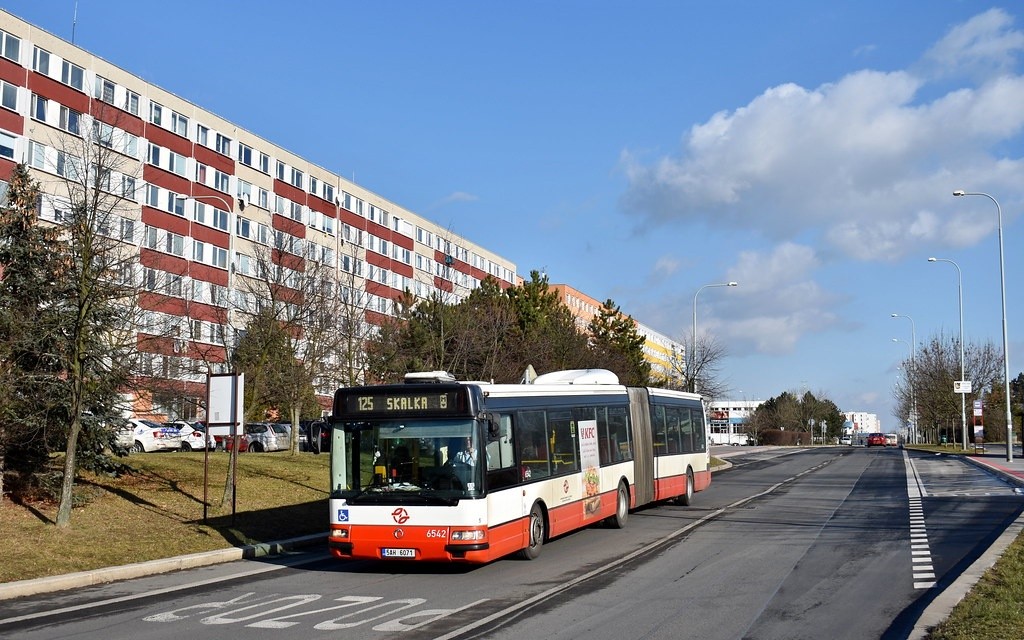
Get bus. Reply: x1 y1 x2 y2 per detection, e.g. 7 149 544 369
307 365 712 564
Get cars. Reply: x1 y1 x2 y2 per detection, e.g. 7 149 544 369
278 422 329 452
866 433 886 447
82 411 136 450
840 437 852 446
884 433 898 445
130 419 184 454
191 421 249 453
244 423 290 453
160 419 217 453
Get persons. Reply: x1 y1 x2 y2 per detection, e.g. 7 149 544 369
454 438 477 466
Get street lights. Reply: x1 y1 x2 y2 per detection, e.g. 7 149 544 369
926 257 969 454
951 189 1013 463
174 188 235 373
891 312 920 447
692 281 740 394
